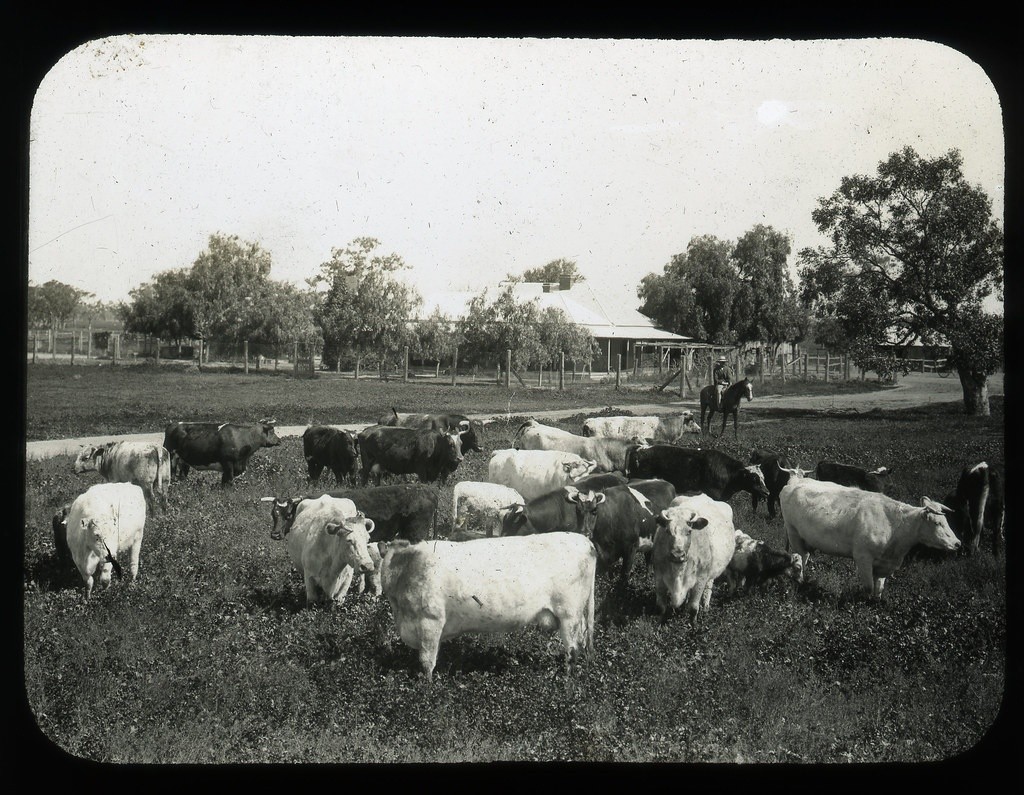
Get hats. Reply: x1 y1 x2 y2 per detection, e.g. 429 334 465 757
715 355 729 362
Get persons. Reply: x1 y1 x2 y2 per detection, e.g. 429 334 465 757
713 355 733 411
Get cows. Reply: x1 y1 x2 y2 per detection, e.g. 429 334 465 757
163 414 282 488
260 409 1002 684
52 481 147 602
73 441 172 516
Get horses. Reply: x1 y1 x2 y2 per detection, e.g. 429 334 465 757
700 376 755 439
